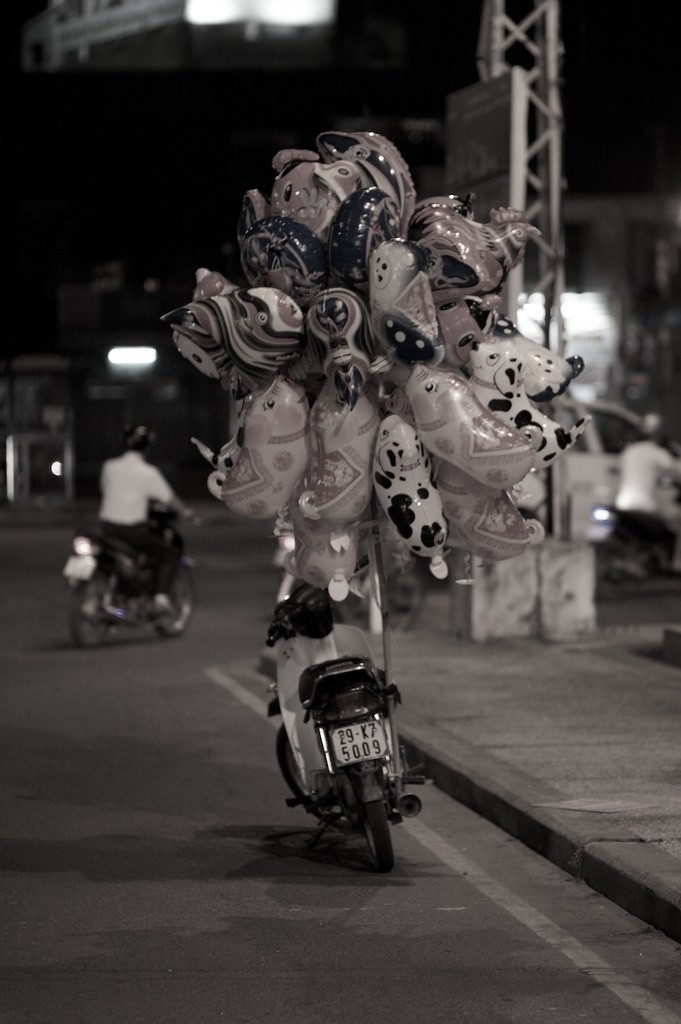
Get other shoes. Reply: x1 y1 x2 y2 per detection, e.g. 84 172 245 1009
154 594 174 612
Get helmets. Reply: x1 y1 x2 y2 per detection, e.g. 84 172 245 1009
641 413 672 439
124 423 158 451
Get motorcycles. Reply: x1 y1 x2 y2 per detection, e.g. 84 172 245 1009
584 503 681 600
266 554 426 873
62 502 197 649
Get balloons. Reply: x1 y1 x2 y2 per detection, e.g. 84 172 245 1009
161 130 594 589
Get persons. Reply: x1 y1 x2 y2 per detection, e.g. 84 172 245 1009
96 427 198 619
609 412 681 573
